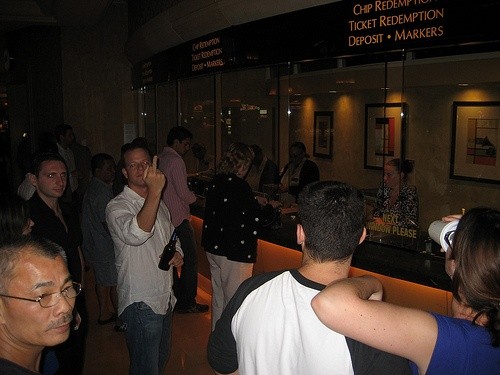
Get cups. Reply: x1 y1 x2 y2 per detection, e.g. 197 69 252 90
283 194 292 208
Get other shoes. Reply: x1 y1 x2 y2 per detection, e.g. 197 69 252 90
115 322 126 332
176 304 209 315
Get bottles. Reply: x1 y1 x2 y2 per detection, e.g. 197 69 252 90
157 229 181 271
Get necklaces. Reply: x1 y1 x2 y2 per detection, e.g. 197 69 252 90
472 310 485 324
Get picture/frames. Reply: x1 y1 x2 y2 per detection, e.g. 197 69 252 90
314 110 333 157
363 102 408 171
448 100 500 184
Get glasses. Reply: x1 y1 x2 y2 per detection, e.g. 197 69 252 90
0 280 81 308
444 230 458 253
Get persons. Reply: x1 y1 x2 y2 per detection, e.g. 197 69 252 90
277 143 318 205
105 145 184 375
200 143 280 331
207 180 411 375
191 144 215 176
153 127 208 314
0 194 60 375
24 154 89 375
369 158 419 231
7 124 154 332
244 147 280 195
0 235 82 375
311 206 500 375
